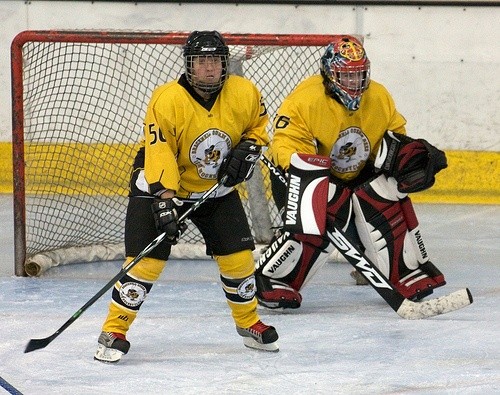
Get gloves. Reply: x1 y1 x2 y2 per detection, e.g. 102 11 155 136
151 198 187 245
218 137 260 187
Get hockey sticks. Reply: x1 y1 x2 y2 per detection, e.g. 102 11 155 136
23 174 228 354
259 156 473 319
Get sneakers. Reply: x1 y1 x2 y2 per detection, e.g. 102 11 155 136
94 331 130 363
235 320 279 352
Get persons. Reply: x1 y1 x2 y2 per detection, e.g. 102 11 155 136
93 31 280 364
254 37 447 311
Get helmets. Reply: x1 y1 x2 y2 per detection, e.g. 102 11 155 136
184 30 230 94
320 38 370 110
